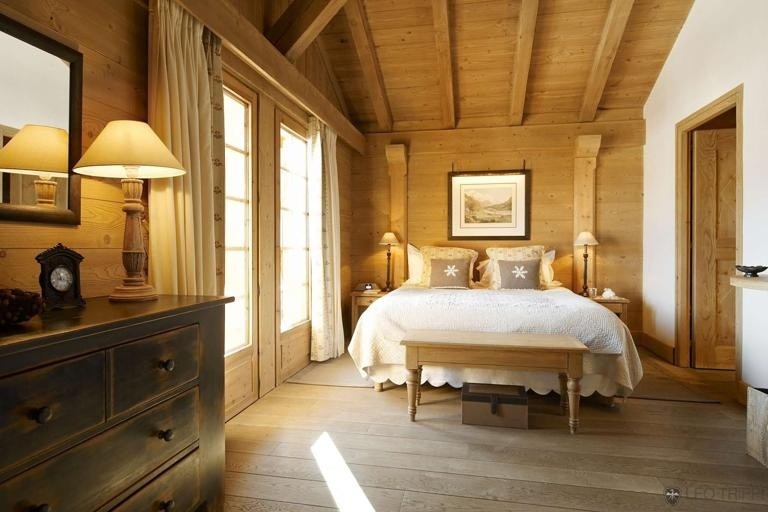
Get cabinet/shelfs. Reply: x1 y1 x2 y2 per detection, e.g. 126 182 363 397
1 296 234 511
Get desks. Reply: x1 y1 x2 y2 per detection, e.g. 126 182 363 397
399 330 590 434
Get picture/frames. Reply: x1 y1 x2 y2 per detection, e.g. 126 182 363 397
447 168 531 241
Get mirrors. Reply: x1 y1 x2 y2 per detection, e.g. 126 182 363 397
0 14 81 226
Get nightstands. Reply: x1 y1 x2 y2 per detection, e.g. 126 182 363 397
592 298 630 324
349 292 388 335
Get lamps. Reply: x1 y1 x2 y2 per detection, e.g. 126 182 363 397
574 233 599 296
0 126 70 208
379 233 400 293
73 120 189 303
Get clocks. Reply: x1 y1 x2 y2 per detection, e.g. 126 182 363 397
36 243 84 306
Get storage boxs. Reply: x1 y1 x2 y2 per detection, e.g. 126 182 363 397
462 383 529 430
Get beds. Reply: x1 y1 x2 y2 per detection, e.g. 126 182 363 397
348 243 643 403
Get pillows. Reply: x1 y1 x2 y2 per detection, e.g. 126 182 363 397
405 243 423 286
476 250 561 287
486 246 546 288
498 260 541 288
421 245 478 290
429 256 471 289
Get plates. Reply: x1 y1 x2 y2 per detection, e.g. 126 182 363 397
736 265 768 278
